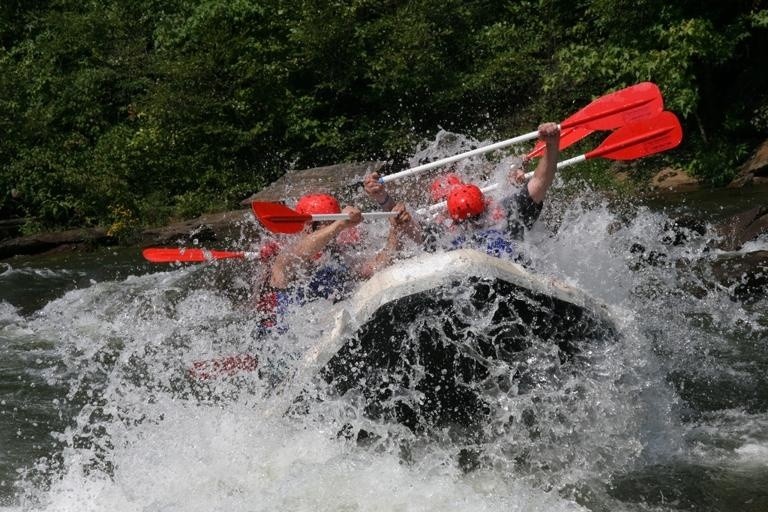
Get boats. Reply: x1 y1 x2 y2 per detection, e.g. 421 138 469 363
253 247 630 477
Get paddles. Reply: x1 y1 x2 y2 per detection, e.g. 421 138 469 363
251 200 401 234
142 248 419 263
479 124 594 189
378 81 663 185
413 111 682 217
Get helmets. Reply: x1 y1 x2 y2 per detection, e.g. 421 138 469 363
295 194 343 233
430 174 460 203
447 185 483 223
